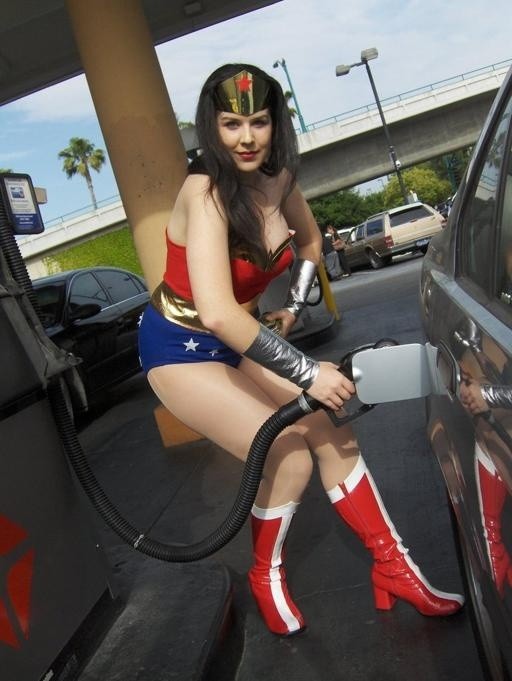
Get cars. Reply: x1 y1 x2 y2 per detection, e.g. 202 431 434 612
419 65 512 681
312 202 447 279
30 266 152 393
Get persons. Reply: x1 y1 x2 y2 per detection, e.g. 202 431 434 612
322 234 345 281
327 225 351 277
461 380 512 601
137 63 466 636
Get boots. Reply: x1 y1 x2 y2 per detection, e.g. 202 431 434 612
474 443 512 601
325 447 465 617
247 502 305 637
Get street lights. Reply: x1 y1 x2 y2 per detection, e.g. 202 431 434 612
335 48 410 203
272 58 308 134
